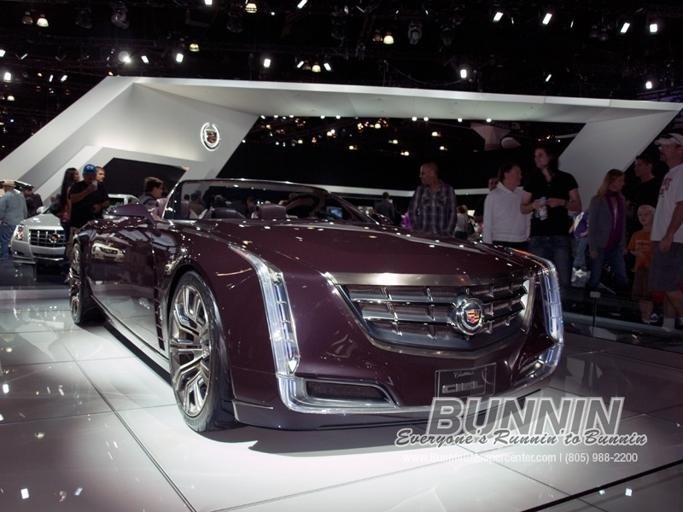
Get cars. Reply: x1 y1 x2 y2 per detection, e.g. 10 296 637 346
10 193 142 274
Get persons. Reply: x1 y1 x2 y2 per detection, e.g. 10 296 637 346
0 165 258 283
375 131 682 338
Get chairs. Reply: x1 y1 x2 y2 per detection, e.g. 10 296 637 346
152 197 288 219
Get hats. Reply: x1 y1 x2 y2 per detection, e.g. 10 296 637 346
82 163 96 176
655 131 682 146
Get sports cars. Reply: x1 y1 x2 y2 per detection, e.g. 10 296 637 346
64 176 568 438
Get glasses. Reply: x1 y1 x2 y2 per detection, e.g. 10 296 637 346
659 133 681 143
